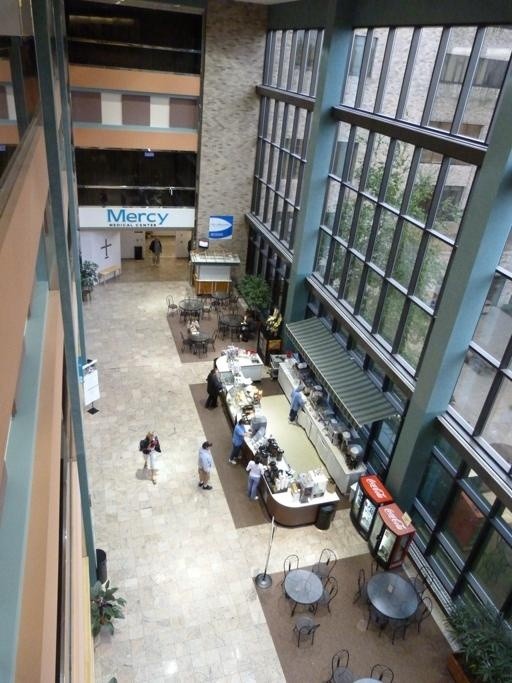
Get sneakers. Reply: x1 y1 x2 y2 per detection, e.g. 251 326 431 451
251 495 259 501
199 480 212 490
228 455 242 464
141 463 158 485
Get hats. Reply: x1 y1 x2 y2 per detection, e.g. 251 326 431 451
238 416 248 425
298 385 306 391
202 441 213 448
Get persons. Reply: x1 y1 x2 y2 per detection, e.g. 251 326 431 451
288 384 307 424
138 430 162 483
203 368 222 410
245 454 264 500
148 235 162 267
229 417 250 464
198 440 213 489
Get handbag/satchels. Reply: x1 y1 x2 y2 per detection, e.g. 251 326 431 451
140 437 162 454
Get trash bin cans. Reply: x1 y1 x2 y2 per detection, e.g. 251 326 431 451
315 503 334 530
135 247 142 259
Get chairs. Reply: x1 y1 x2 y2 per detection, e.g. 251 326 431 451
280 548 434 683
166 283 247 359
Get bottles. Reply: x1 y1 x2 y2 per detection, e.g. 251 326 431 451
291 482 298 500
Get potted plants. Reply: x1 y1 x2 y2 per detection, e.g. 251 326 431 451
90 579 125 637
237 275 273 334
443 595 512 683
80 259 99 302
